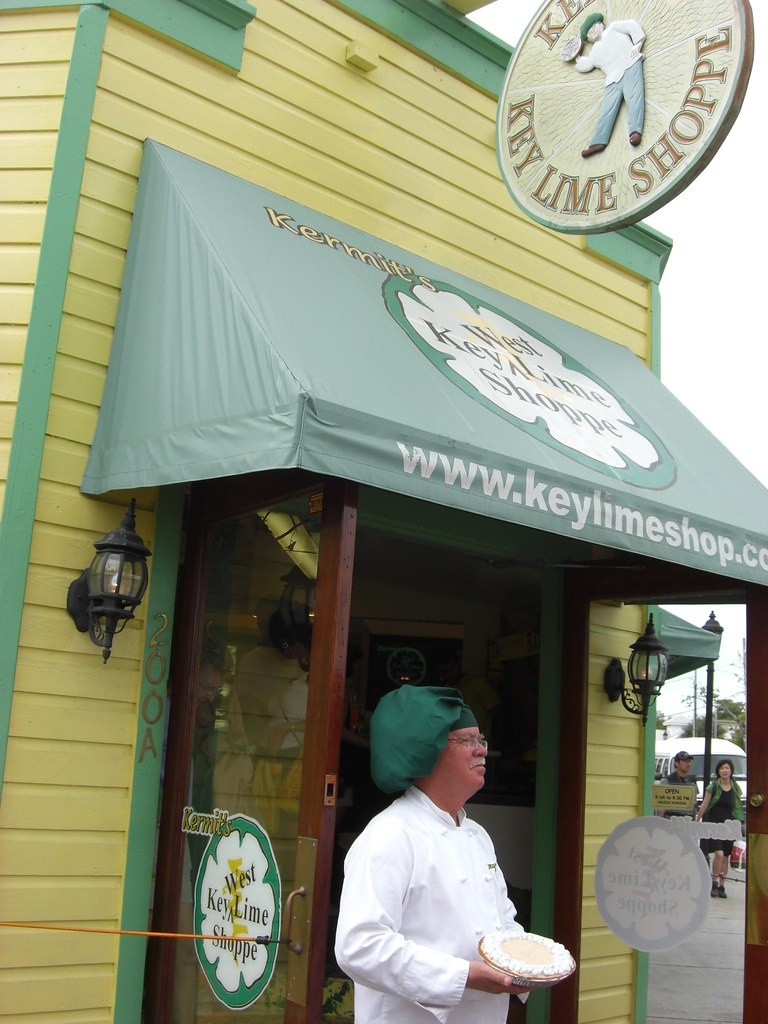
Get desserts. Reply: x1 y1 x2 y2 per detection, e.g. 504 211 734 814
481 931 574 978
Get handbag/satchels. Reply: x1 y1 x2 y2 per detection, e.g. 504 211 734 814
729 840 746 873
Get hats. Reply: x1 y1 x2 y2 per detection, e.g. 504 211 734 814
370 684 479 792
675 751 694 760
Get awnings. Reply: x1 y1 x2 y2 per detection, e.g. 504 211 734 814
78 137 768 606
659 606 721 680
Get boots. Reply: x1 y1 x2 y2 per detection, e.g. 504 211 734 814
710 881 719 896
718 886 727 898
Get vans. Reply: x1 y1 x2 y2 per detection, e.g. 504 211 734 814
653 736 747 823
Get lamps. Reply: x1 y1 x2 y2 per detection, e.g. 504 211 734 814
269 560 316 684
602 612 665 728
65 498 151 663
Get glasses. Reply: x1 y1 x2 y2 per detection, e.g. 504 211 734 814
447 733 488 754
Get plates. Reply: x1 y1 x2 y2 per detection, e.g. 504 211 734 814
479 954 576 988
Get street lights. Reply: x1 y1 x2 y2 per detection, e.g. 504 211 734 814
700 611 726 804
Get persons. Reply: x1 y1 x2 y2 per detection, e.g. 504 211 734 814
574 13 647 158
658 751 701 822
697 759 745 898
334 684 538 1024
